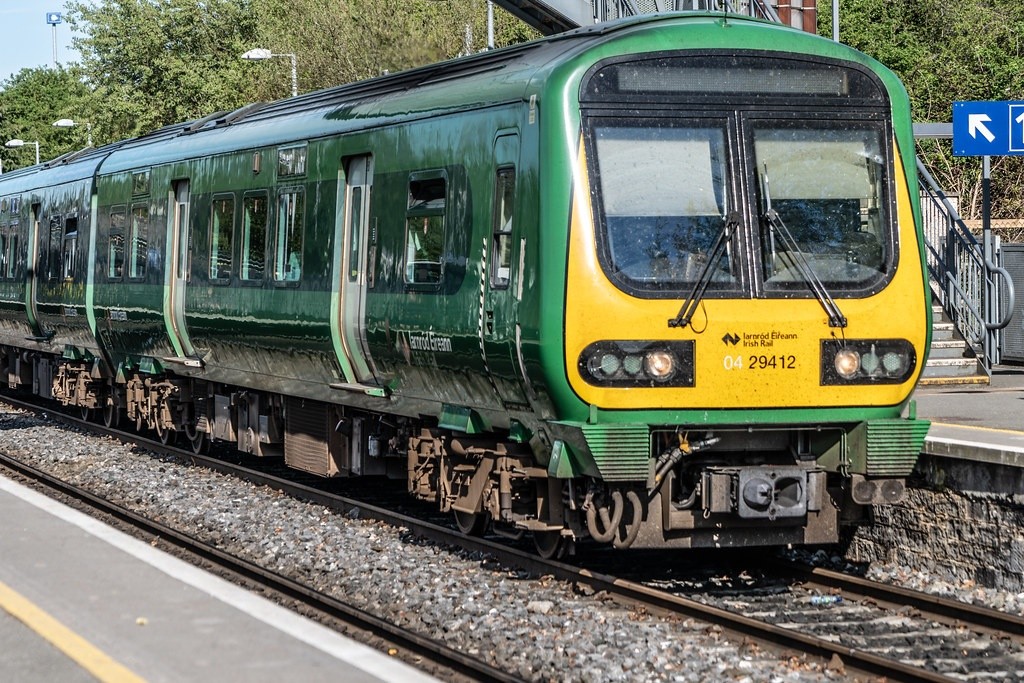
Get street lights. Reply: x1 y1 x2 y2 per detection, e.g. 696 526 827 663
52 118 93 146
5 139 39 165
240 48 298 97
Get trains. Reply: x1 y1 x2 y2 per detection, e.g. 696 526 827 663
0 10 930 568
